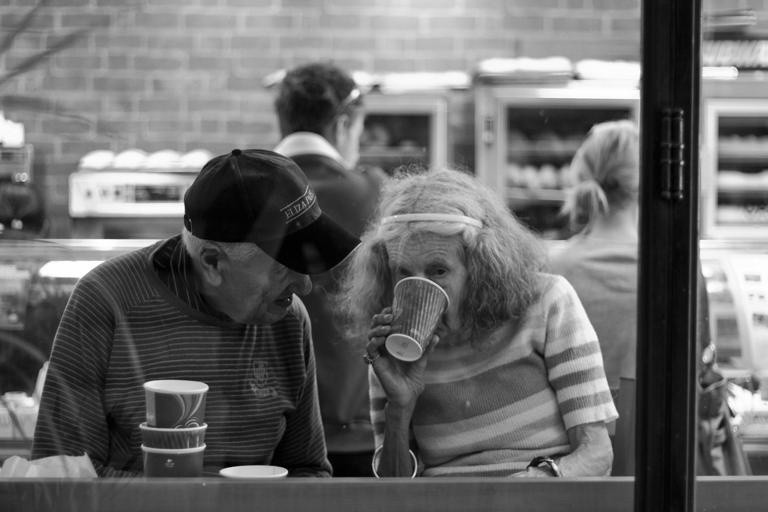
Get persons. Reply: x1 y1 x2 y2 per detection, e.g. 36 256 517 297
540 118 711 475
331 165 621 480
28 147 363 476
268 61 394 475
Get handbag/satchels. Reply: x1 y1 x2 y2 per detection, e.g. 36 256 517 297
694 281 756 482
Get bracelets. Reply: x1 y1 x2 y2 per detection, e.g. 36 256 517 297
525 454 563 477
371 442 418 479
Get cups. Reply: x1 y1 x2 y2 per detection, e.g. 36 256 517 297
384 275 450 362
140 379 209 477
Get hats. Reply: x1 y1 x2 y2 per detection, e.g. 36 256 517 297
183 148 365 276
